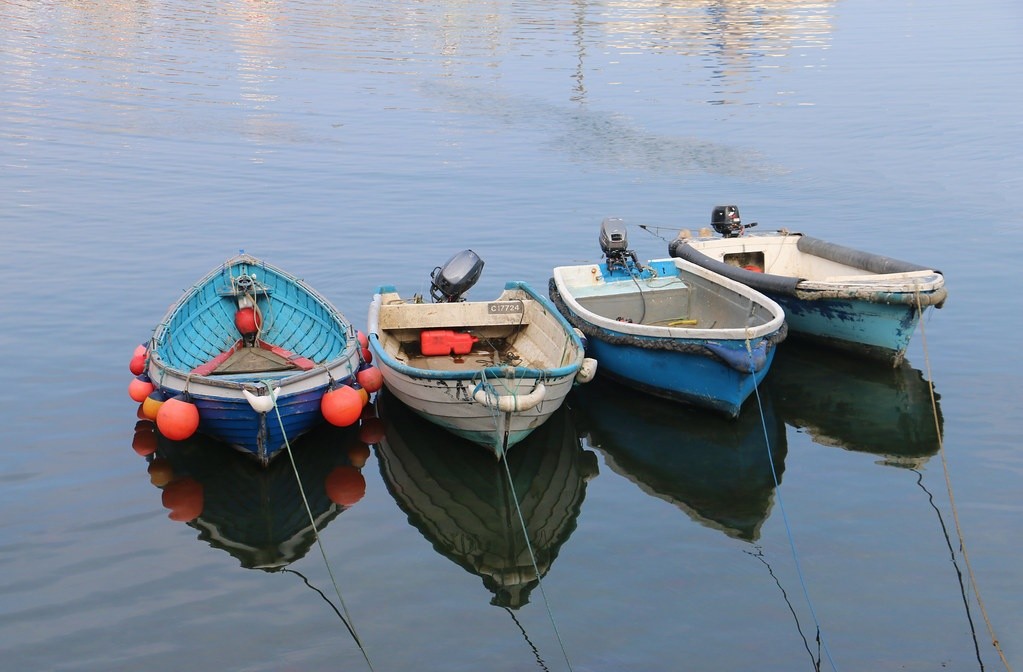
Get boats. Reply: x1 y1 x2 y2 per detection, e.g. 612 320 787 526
585 367 788 542
371 382 588 610
151 399 371 572
773 341 944 469
548 219 788 421
146 253 364 468
668 206 948 367
367 249 585 460
